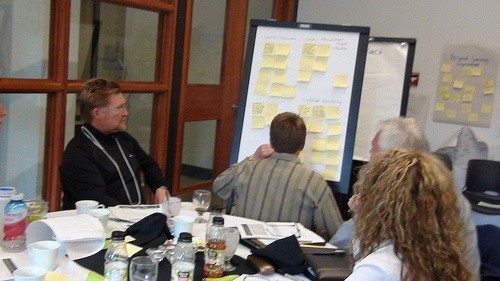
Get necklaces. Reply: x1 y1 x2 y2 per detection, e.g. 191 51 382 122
81 126 141 205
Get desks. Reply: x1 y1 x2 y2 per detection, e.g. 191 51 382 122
0 208 341 281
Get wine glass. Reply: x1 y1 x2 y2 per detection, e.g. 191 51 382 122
223 228 240 272
193 190 212 223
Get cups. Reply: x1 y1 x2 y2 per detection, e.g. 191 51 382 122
162 196 181 218
75 200 105 216
88 209 110 231
27 241 61 272
13 266 45 281
168 215 195 240
129 256 159 281
0 186 16 246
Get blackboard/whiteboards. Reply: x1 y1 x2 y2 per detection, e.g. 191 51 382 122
230 19 370 194
351 37 416 166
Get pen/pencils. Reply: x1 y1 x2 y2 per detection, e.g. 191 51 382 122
119 206 159 209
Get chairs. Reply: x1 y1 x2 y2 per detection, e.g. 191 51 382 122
462 158 500 216
474 224 500 281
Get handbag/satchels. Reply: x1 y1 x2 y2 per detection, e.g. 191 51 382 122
111 212 173 248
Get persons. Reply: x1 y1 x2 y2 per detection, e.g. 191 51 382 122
59 77 169 209
344 147 472 281
213 112 342 237
330 117 481 281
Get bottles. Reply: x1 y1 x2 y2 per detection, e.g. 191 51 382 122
2 193 27 252
104 231 129 281
204 216 226 278
171 232 196 281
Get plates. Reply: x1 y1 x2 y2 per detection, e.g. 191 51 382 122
64 237 105 260
